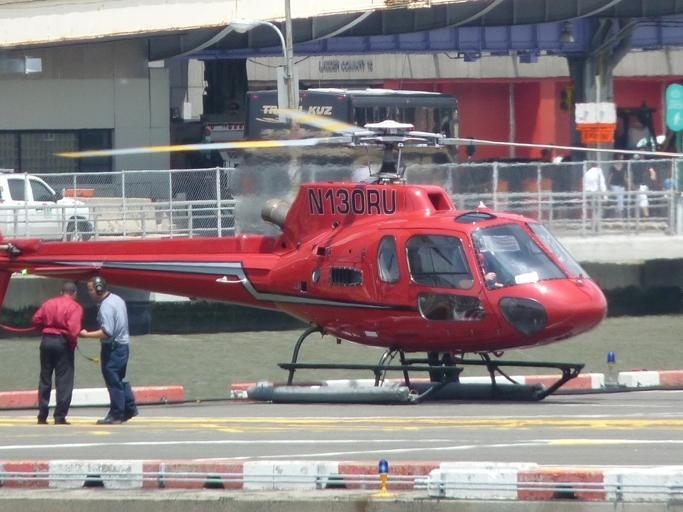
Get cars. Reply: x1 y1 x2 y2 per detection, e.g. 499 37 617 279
0 173 94 242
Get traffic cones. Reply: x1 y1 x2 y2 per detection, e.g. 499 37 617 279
0 459 682 506
0 384 186 410
618 369 681 390
230 372 606 398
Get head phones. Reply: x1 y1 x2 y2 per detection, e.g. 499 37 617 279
94 276 105 296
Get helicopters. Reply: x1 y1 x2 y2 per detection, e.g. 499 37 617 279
0 119 683 405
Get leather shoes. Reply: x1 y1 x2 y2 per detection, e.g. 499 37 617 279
97 412 123 425
55 417 73 425
123 408 139 422
38 419 49 424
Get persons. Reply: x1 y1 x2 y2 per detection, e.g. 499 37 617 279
583 161 608 231
631 147 656 217
33 282 83 425
80 277 139 425
608 153 628 218
663 170 676 197
454 254 497 320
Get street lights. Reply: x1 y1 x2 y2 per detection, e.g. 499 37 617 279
226 15 299 129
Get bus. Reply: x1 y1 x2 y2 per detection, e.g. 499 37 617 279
243 88 458 164
